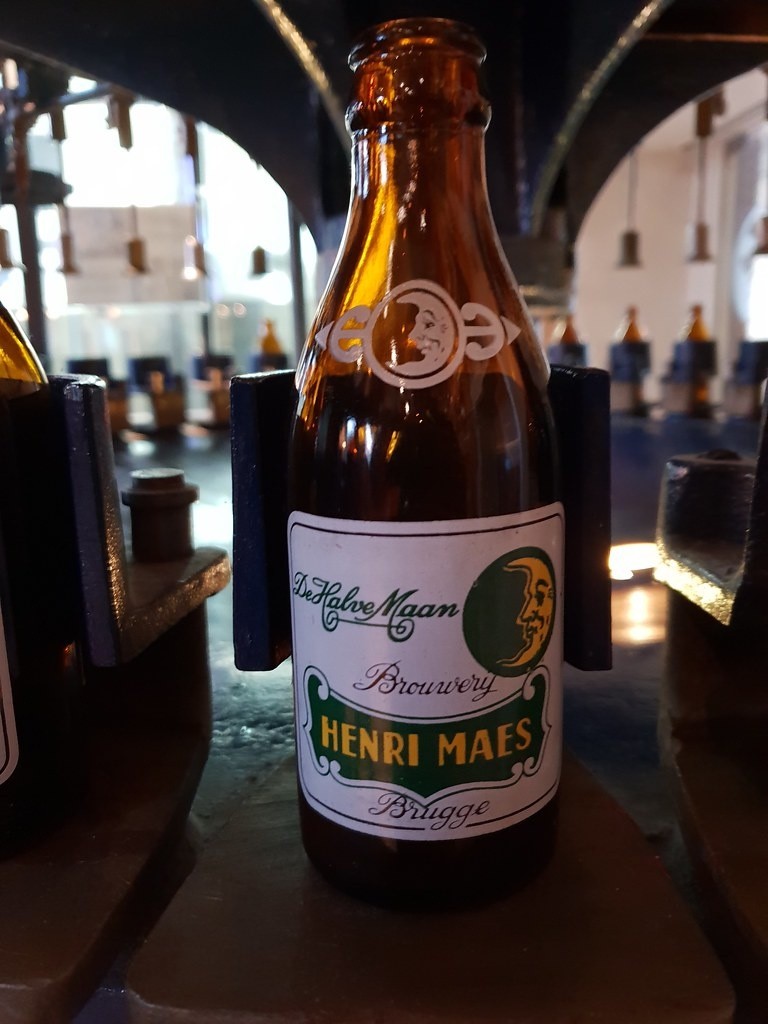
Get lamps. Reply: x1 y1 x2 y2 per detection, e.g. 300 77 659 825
56 146 78 274
0 229 16 271
251 246 268 276
752 132 768 255
124 202 149 275
616 152 643 268
688 140 712 263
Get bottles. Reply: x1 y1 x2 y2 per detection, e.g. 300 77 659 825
551 314 577 346
0 301 85 852
613 309 642 411
260 322 277 355
280 16 564 902
679 306 707 416
